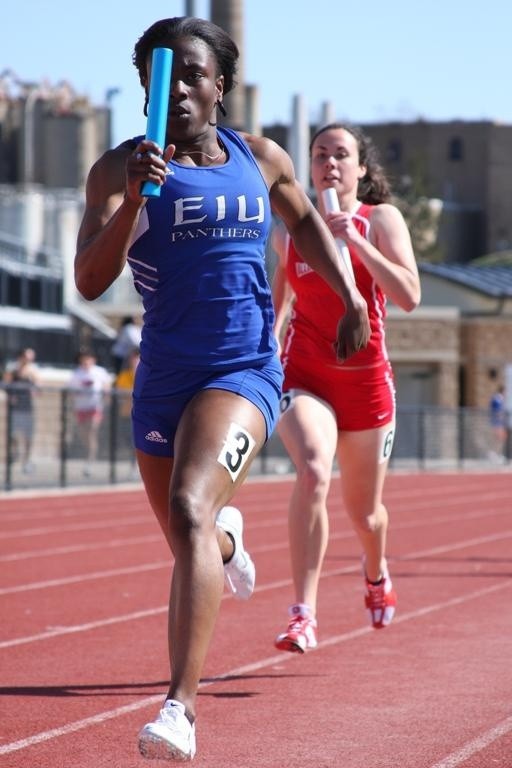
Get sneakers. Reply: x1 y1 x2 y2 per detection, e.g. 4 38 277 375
275 605 319 653
215 507 255 601
362 556 398 629
138 700 196 758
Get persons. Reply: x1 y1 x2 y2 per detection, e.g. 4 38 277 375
75 19 373 762
270 122 422 652
2 349 44 475
74 347 140 478
487 381 507 463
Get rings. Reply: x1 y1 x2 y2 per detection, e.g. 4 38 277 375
136 153 141 162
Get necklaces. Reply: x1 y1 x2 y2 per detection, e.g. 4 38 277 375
173 134 224 160
